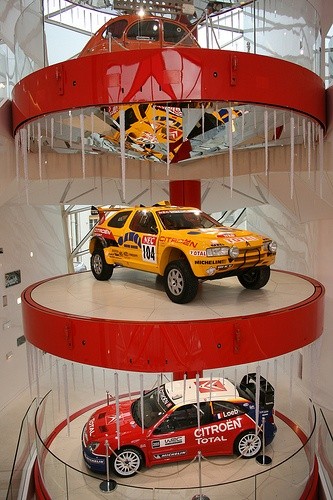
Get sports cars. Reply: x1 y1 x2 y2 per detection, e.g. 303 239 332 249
86 200 279 303
77 15 208 53
77 369 279 478
